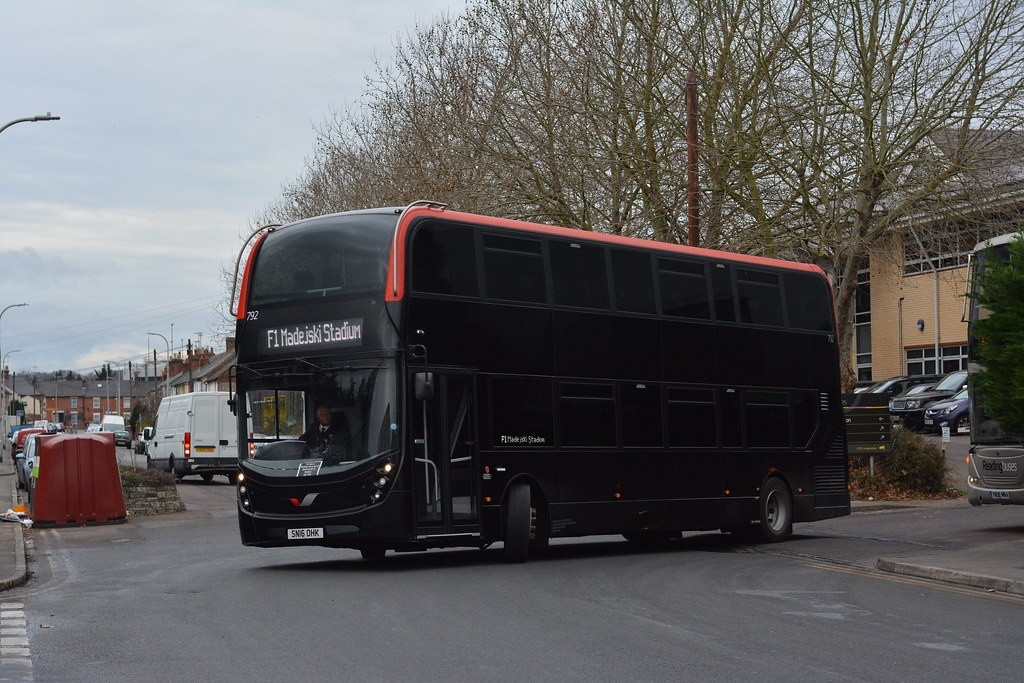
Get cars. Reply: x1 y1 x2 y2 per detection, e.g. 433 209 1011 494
13 419 153 504
842 369 971 437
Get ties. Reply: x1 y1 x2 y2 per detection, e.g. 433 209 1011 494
322 427 325 433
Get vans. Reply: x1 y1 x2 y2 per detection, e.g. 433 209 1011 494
144 391 254 486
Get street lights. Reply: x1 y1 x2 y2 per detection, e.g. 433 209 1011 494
148 332 169 396
55 378 67 419
2 350 22 449
106 359 120 416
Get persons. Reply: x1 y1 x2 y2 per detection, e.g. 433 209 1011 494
298 405 352 459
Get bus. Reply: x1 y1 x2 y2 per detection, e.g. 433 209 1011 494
960 231 1024 506
227 198 852 564
